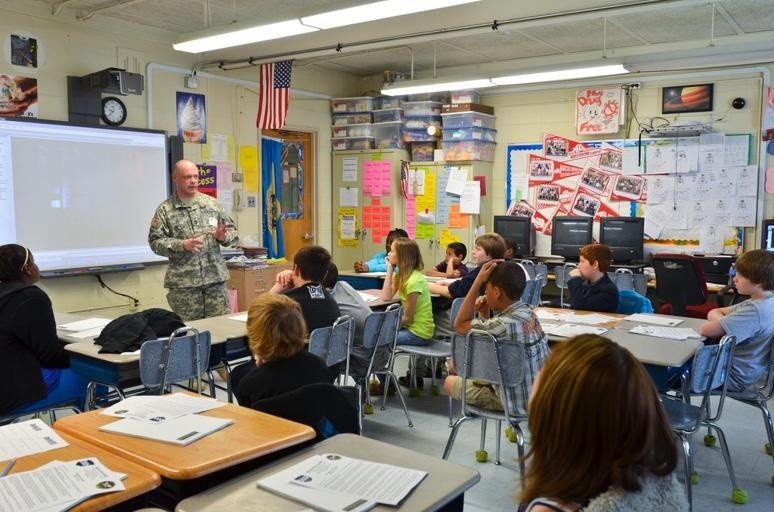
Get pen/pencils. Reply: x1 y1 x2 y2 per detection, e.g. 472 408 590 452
0 458 15 476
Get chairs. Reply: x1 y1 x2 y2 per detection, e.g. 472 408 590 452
516 253 736 321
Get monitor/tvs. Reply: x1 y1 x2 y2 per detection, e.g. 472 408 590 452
761 219 774 253
551 216 593 263
600 217 644 265
494 216 536 259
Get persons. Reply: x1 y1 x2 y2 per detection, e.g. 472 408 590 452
512 333 689 512
444 260 552 416
148 160 238 322
567 243 619 312
0 243 90 416
228 293 330 404
674 249 774 398
352 228 516 391
269 246 388 384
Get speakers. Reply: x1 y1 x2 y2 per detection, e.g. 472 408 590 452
169 135 183 193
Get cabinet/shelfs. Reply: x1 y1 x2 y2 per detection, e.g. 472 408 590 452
403 163 492 270
226 263 293 312
331 149 408 273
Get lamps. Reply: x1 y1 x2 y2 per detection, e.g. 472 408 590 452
172 1 482 54
381 21 630 97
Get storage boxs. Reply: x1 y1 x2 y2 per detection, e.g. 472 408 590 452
401 100 442 161
371 95 404 149
441 89 497 161
331 96 374 150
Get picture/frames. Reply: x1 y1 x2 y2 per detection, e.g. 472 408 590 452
662 83 713 114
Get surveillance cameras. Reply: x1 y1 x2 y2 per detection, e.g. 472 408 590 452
732 97 745 109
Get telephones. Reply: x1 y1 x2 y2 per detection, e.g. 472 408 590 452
234 188 244 211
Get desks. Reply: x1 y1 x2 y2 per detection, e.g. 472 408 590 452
335 265 480 310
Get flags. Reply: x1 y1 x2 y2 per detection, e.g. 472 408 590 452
256 59 292 129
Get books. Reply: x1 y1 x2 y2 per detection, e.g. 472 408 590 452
536 310 702 340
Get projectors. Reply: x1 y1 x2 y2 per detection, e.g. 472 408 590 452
80 67 145 96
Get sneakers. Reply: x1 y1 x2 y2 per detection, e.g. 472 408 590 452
399 376 424 388
373 383 396 394
425 369 441 378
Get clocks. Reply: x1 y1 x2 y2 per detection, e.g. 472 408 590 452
101 97 127 126
